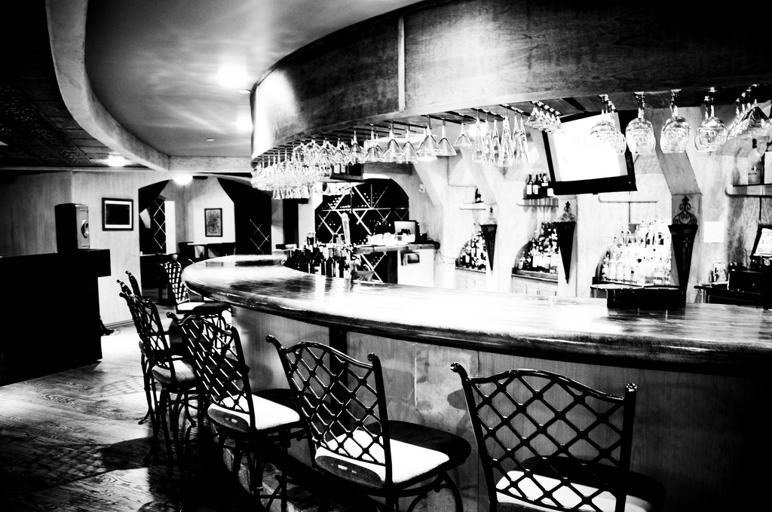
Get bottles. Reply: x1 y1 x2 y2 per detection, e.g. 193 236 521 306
526 172 554 200
474 188 481 203
455 230 487 271
519 222 559 273
301 246 350 279
747 137 763 184
561 200 576 222
603 228 668 285
487 205 497 224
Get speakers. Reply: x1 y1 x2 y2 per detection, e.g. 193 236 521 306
55 203 90 251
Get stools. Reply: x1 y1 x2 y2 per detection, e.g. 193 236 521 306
265 332 471 512
166 312 333 512
119 291 246 463
116 280 251 440
117 271 199 428
450 359 657 512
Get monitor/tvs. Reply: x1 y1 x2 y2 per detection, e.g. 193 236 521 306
542 110 637 195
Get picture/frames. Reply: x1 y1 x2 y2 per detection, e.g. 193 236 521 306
204 207 223 237
102 196 134 230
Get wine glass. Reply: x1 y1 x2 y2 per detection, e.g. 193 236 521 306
589 83 768 157
525 105 563 136
250 115 458 200
